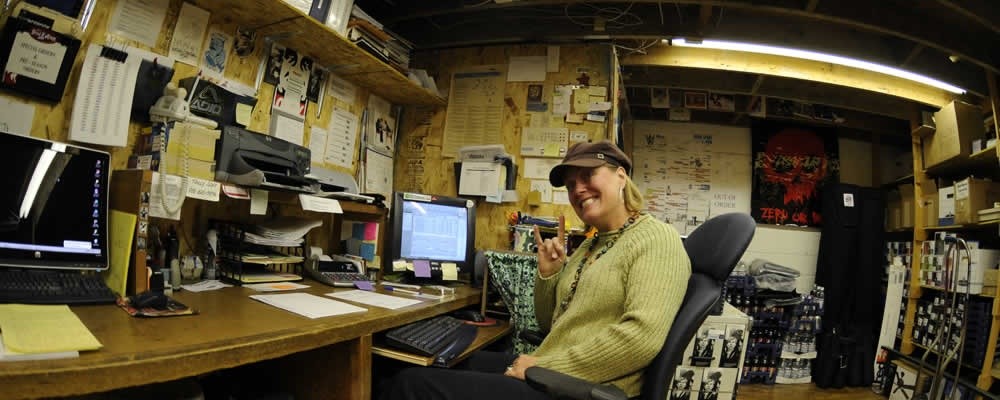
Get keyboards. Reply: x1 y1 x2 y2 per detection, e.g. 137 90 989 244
374 316 478 360
0 268 117 305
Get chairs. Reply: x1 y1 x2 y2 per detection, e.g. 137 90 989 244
526 211 756 400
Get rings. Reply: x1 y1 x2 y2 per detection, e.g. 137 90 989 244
506 365 515 369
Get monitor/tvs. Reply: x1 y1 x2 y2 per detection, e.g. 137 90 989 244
386 191 475 287
0 132 110 272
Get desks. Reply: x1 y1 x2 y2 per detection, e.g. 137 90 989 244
110 168 390 295
0 282 513 400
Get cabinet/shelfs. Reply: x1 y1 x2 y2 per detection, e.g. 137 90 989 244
879 114 1000 400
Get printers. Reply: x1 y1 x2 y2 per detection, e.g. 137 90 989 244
213 125 318 195
309 166 375 204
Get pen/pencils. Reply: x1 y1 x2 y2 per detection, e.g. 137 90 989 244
384 286 422 295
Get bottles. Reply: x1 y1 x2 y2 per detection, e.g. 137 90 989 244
722 260 826 385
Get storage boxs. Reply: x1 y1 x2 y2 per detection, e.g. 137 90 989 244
912 98 1000 294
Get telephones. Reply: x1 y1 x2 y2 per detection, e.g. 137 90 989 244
149 95 218 130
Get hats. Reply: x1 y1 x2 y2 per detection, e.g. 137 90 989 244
550 139 632 187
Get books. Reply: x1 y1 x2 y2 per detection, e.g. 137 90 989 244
285 0 409 78
106 210 137 297
129 112 222 184
0 303 104 362
216 215 424 320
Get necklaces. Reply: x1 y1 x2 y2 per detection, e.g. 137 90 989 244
562 213 644 311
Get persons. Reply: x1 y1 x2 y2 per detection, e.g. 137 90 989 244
378 140 691 400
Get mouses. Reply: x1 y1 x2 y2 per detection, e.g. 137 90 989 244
130 290 169 311
454 310 485 322
